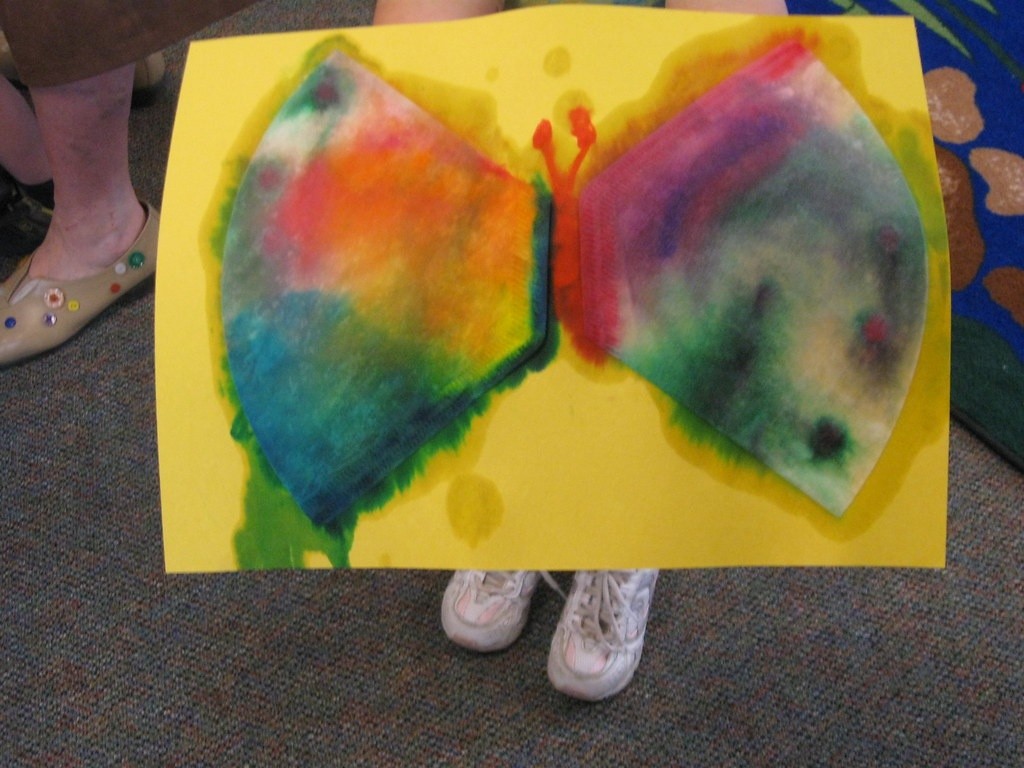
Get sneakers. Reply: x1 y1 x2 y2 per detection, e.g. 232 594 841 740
442 570 567 651
547 569 658 700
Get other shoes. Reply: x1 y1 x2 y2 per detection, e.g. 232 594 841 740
0 186 53 256
131 50 170 103
0 199 159 362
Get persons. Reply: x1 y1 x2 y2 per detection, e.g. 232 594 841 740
370 0 802 704
0 0 260 368
